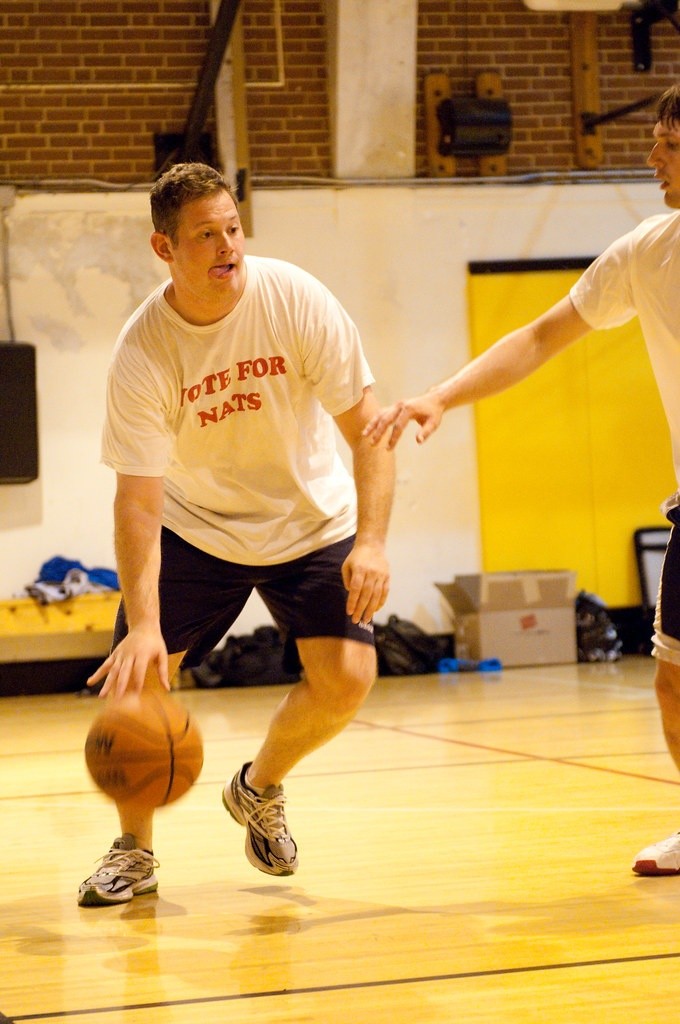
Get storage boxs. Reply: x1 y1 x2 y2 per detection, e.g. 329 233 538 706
435 568 578 669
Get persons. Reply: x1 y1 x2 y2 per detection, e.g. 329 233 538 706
74 162 397 906
363 86 680 879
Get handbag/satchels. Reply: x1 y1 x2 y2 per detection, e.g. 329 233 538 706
570 590 622 661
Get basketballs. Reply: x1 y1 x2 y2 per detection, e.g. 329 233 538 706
84 690 204 805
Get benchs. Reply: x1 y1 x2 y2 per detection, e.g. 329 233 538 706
0 591 179 693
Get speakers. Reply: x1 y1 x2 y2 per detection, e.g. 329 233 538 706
0 341 39 485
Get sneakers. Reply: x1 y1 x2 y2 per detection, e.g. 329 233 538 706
630 832 679 877
221 761 299 876
76 834 160 905
